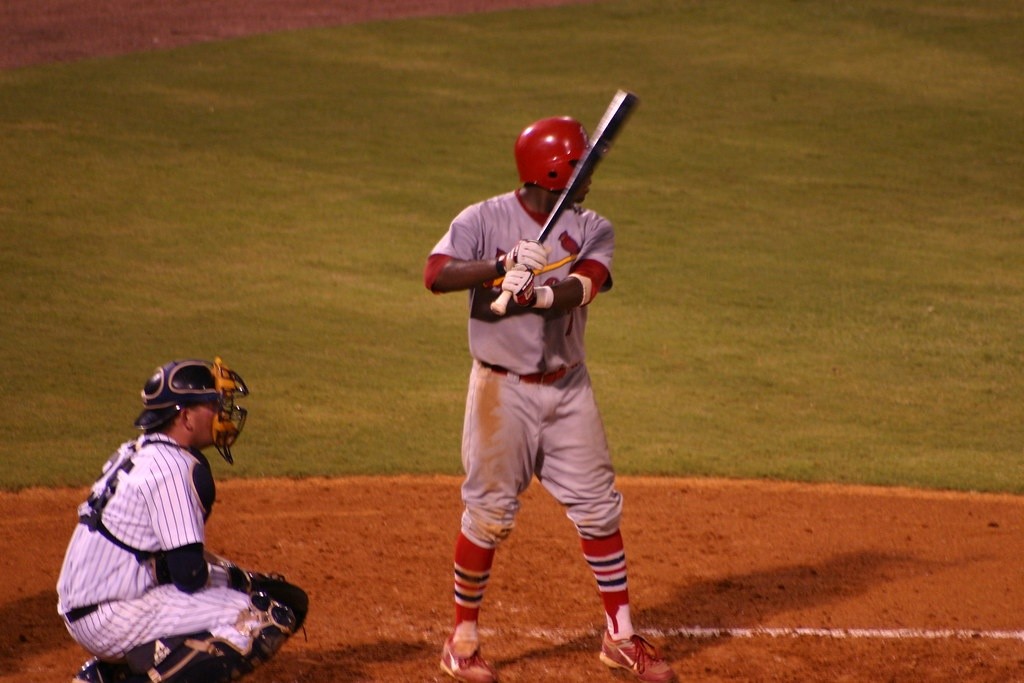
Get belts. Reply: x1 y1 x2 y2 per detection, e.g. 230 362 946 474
480 361 579 386
65 603 98 623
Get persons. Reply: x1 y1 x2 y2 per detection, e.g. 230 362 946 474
56 358 308 683
424 117 673 683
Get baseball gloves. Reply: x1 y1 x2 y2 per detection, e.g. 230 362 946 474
229 563 311 635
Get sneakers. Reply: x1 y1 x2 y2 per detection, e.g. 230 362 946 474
599 629 676 683
440 635 499 683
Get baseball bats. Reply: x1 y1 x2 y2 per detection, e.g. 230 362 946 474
487 93 639 317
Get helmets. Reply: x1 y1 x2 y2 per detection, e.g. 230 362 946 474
133 359 219 430
514 117 589 192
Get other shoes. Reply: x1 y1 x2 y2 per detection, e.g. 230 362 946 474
70 656 134 683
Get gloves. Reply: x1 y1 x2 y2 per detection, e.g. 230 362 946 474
500 264 555 309
495 239 552 276
